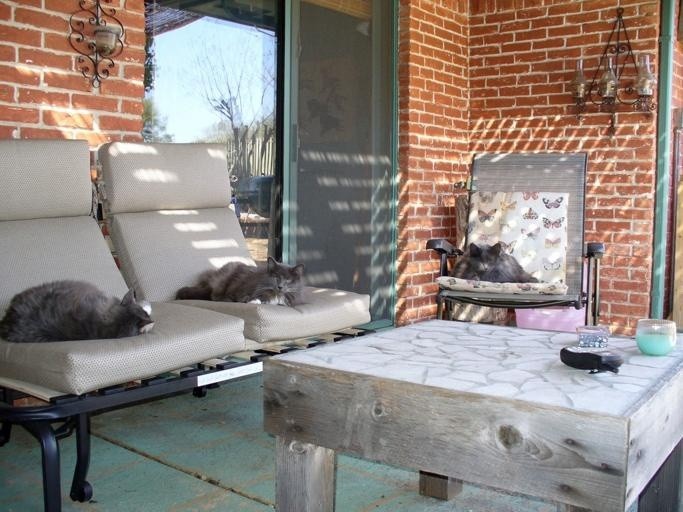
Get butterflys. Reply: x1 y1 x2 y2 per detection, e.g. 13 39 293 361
468 192 565 284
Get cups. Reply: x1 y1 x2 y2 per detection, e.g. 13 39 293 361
636 317 678 357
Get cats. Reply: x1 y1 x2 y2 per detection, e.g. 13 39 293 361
175 256 305 309
450 242 539 283
0 280 156 343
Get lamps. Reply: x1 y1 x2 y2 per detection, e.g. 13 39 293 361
569 8 659 118
70 3 126 92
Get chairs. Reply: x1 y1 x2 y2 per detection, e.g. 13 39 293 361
425 150 603 329
0 141 376 508
